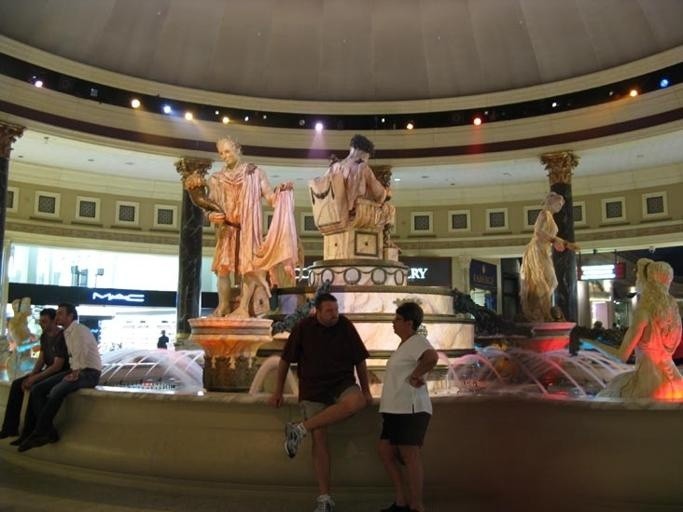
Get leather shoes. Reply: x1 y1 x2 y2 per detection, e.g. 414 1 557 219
0 428 47 452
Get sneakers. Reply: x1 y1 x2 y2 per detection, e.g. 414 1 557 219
381 501 424 511
314 492 335 512
283 422 305 459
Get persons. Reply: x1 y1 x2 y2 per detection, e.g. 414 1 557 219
268 293 373 512
204 135 292 319
580 258 683 399
17 304 101 452
518 192 566 322
378 302 439 512
157 330 169 350
0 308 70 446
325 134 398 248
